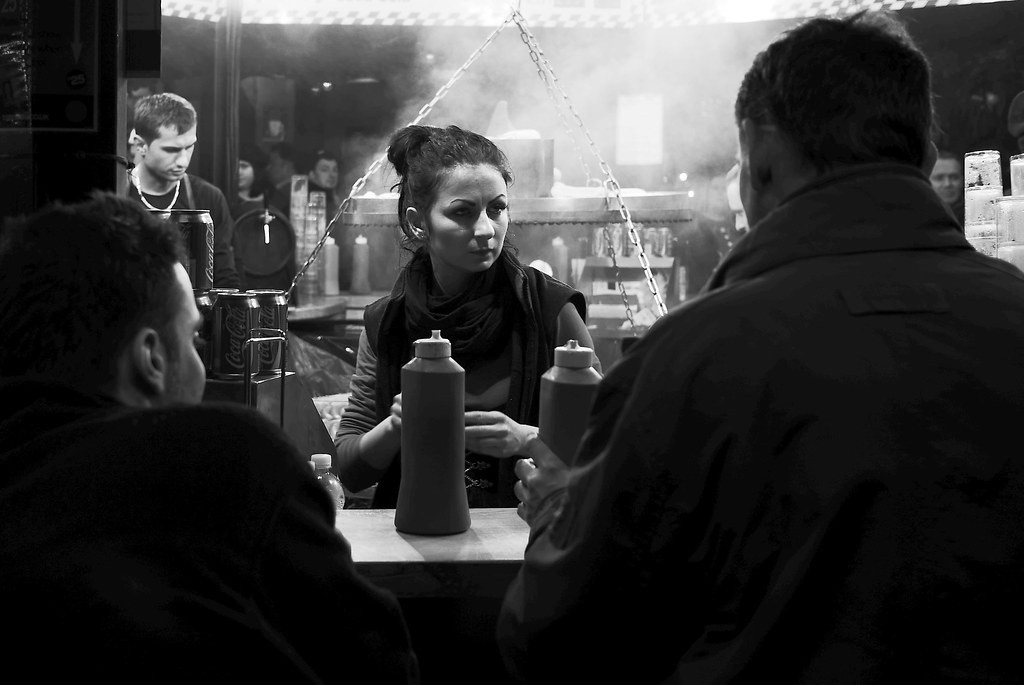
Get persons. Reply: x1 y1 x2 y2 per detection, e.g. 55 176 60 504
1 190 421 684
490 10 1023 685
333 126 603 685
123 94 344 399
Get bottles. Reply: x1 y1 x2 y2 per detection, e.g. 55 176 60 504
538 339 601 456
306 461 315 472
350 235 370 294
325 238 341 296
392 331 472 535
552 235 568 284
311 453 346 510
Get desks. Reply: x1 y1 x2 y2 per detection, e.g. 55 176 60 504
335 508 530 561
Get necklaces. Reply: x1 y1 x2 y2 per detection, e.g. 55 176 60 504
136 177 180 222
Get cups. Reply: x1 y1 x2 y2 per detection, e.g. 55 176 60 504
289 175 325 297
965 151 1024 274
